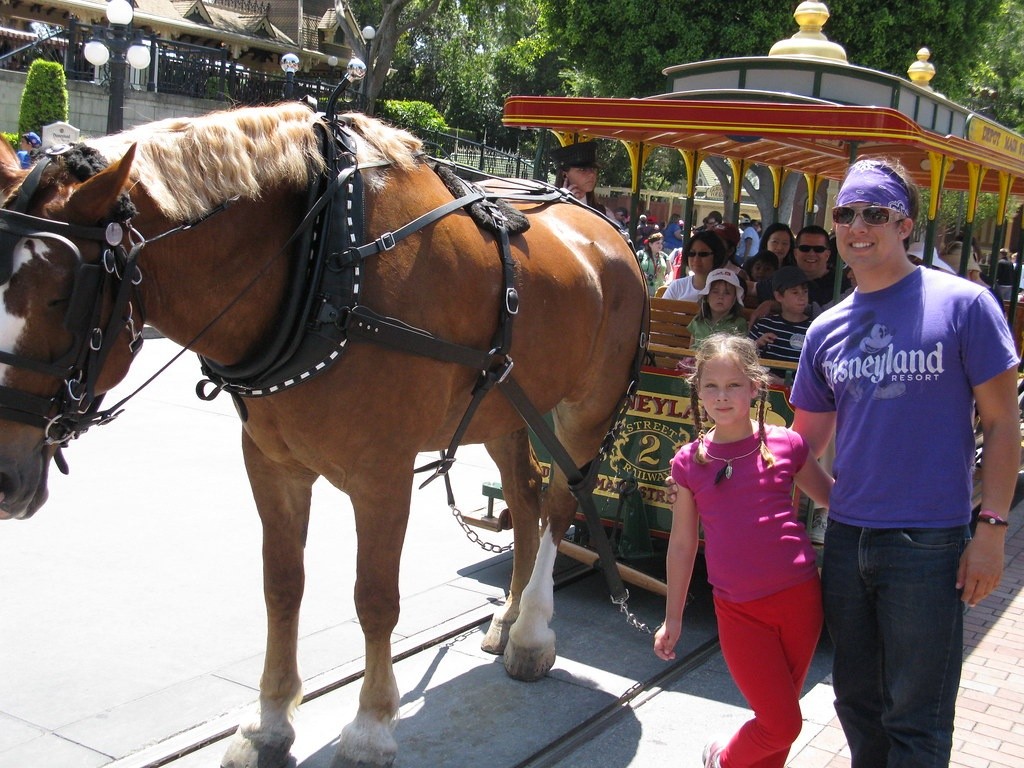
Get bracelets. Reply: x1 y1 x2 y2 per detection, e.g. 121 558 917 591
975 509 1010 528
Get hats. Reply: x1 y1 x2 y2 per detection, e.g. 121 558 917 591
639 214 648 222
906 242 940 266
709 222 740 244
550 142 602 169
771 266 813 291
648 217 656 223
697 268 745 308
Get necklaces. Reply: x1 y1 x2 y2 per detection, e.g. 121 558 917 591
704 418 761 485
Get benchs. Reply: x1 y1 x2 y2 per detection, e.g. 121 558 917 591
650 297 756 370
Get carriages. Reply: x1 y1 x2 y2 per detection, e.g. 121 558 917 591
0 5 1024 766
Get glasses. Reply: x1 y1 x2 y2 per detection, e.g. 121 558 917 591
832 206 907 226
799 244 832 253
687 251 715 257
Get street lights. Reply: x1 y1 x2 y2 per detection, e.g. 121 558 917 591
83 0 151 137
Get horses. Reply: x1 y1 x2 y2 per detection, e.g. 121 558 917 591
0 101 652 768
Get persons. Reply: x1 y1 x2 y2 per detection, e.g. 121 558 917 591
551 141 616 222
613 207 857 386
16 133 41 170
654 333 836 768
665 159 1022 768
907 235 1024 310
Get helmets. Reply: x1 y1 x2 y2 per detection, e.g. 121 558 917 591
22 132 42 148
738 214 751 224
